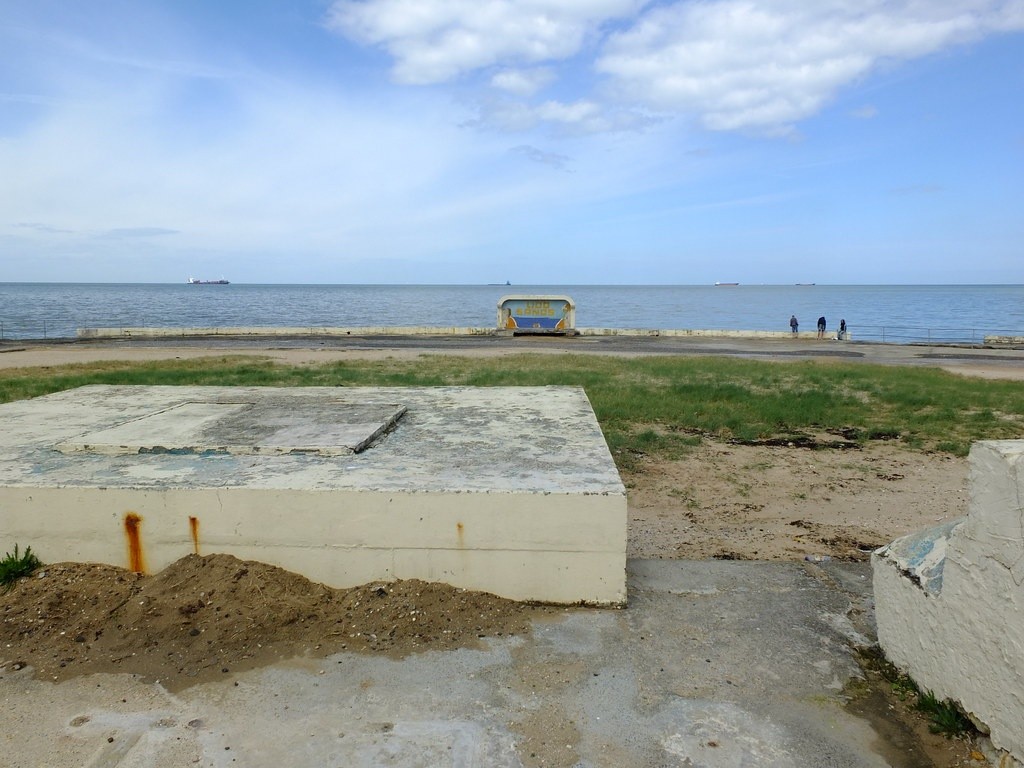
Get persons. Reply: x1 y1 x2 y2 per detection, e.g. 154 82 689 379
816 317 826 340
837 319 847 340
790 315 799 332
